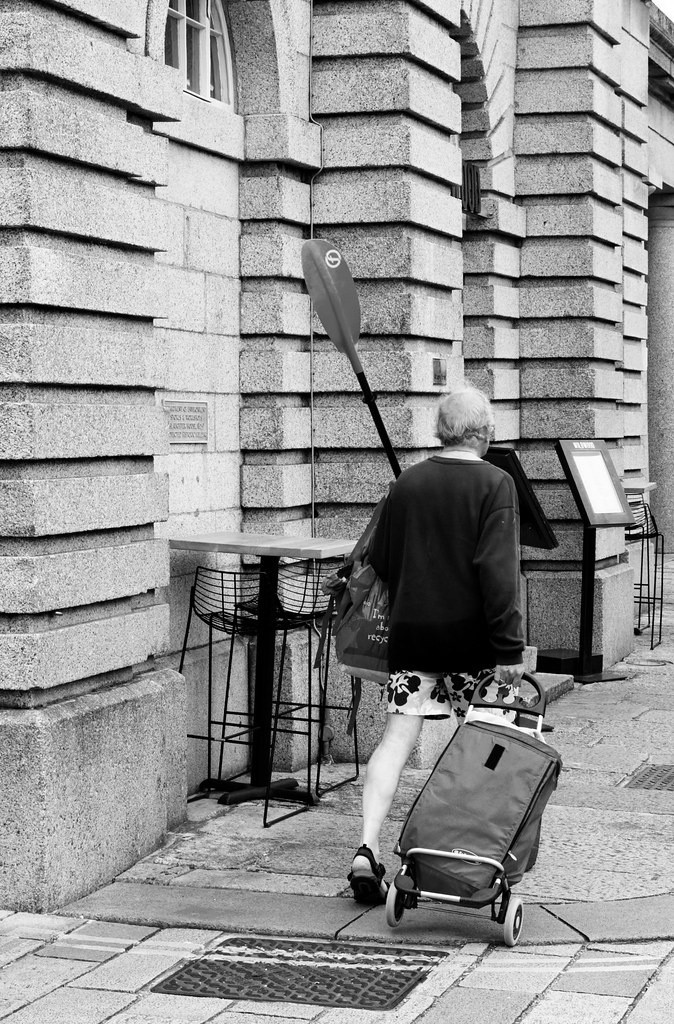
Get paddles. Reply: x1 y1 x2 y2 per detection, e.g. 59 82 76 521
301 240 403 479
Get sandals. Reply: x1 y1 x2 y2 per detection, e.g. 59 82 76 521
347 843 390 907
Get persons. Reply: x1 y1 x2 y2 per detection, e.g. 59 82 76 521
345 390 526 906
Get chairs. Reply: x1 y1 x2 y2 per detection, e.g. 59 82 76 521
625 493 665 650
179 558 359 827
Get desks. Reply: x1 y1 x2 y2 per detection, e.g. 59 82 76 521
168 534 358 804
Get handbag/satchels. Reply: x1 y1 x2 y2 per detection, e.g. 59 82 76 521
313 481 391 685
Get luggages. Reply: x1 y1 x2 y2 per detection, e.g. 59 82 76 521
383 670 563 946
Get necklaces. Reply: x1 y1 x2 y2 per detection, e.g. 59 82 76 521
441 449 475 454
